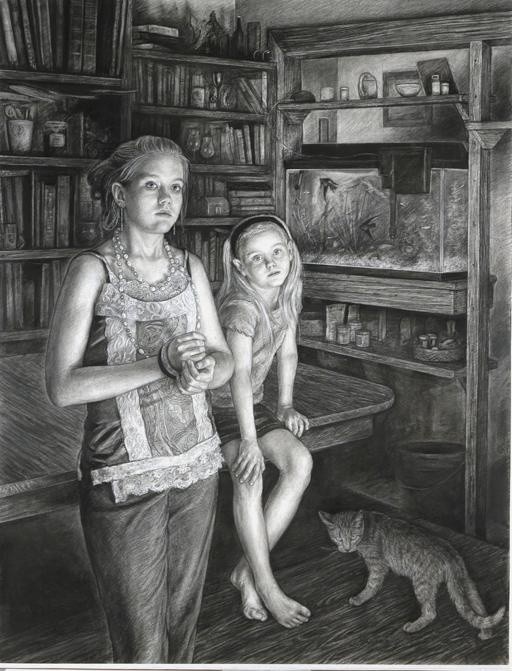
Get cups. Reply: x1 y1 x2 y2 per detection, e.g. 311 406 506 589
320 87 335 102
358 72 378 100
6 119 34 157
78 220 99 246
340 86 349 100
43 120 69 158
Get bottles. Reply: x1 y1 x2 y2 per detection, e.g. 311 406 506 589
232 15 244 59
336 319 371 348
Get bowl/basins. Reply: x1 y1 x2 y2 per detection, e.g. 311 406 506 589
395 83 422 97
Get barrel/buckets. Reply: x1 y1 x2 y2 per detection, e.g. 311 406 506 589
391 438 466 531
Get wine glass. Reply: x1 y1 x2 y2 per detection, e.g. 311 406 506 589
200 136 215 164
212 71 224 111
187 128 201 164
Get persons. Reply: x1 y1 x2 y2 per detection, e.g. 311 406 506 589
205 214 314 629
45 135 234 663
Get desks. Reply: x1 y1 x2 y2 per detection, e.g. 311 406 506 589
0 352 394 524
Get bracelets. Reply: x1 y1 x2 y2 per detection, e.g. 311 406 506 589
158 338 182 380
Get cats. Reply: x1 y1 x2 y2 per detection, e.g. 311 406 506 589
318 509 506 641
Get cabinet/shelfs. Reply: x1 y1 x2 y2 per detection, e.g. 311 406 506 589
0 0 275 342
267 9 511 564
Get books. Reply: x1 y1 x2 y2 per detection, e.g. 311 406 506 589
0 0 275 333
